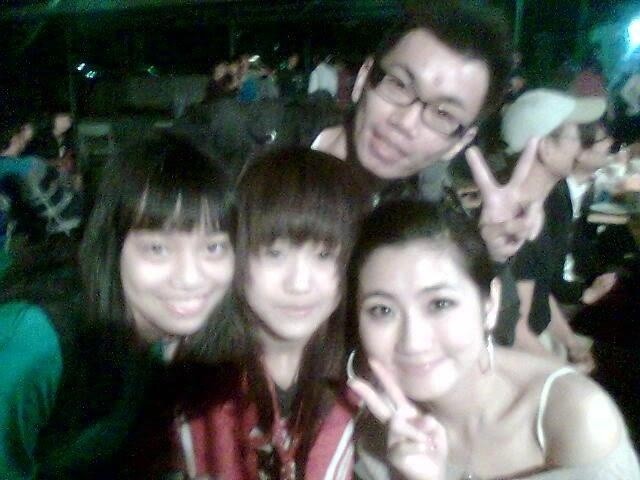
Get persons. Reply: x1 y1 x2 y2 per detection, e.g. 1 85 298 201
2 0 640 480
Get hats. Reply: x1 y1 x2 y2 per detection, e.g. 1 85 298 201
499 87 610 156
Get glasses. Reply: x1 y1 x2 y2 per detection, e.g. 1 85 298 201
589 130 614 146
366 62 469 139
